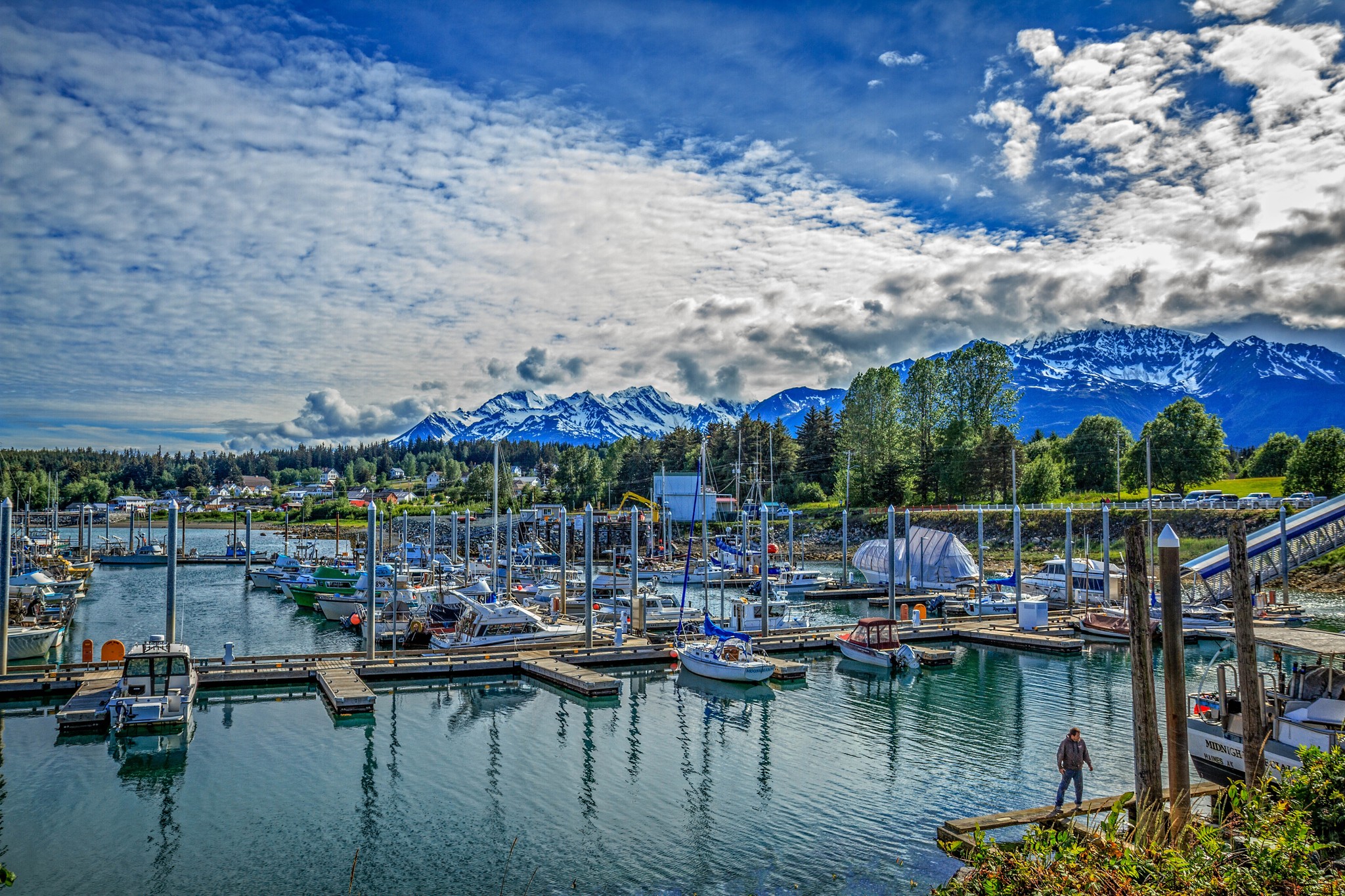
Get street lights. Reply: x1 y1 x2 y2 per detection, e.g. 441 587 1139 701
846 465 860 511
978 544 990 621
801 534 810 570
719 549 732 629
390 557 404 657
1109 449 1121 503
919 536 929 592
600 548 618 632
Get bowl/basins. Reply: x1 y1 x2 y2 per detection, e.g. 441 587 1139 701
41 615 53 622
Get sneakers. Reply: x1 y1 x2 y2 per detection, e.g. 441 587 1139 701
1076 804 1082 810
1054 804 1064 813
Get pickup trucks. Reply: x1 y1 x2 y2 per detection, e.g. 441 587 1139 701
776 508 802 519
1280 491 1328 509
1237 492 1285 510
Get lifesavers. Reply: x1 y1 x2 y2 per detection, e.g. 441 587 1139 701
410 620 423 633
414 586 421 588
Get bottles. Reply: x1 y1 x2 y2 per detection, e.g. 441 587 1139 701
124 690 129 697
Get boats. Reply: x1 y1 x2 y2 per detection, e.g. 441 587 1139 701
179 434 834 657
1019 521 1161 606
1076 554 1164 643
0 470 168 662
852 525 980 591
925 582 996 616
833 616 924 669
1216 588 1317 623
1186 626 1345 806
108 576 199 733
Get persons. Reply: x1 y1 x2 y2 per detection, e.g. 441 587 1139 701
1054 727 1094 814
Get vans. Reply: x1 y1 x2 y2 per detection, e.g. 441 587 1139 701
1181 490 1223 508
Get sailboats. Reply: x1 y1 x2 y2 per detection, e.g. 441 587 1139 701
1093 436 1237 642
962 449 1048 617
667 433 777 683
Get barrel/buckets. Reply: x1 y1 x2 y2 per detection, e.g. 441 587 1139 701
168 688 181 696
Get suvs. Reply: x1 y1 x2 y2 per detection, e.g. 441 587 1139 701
1141 493 1184 509
1201 494 1239 509
